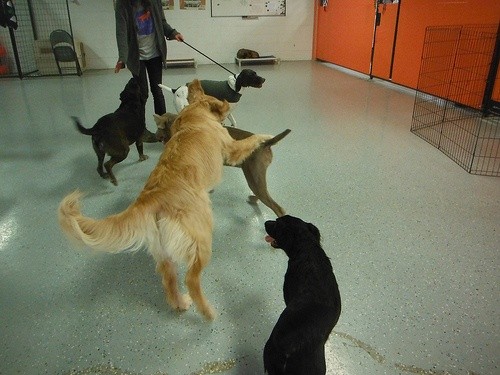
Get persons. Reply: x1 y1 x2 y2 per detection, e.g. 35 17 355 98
113 0 184 143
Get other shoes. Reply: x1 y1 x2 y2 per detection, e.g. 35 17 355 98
141 128 159 143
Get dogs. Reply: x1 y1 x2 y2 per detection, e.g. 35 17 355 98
70 78 158 186
237 49 259 59
157 69 265 128
264 215 341 375
57 79 277 321
152 105 300 217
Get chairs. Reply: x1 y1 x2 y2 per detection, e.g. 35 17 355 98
50 29 82 75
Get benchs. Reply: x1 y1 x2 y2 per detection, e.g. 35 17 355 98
235 55 280 67
162 58 197 68
